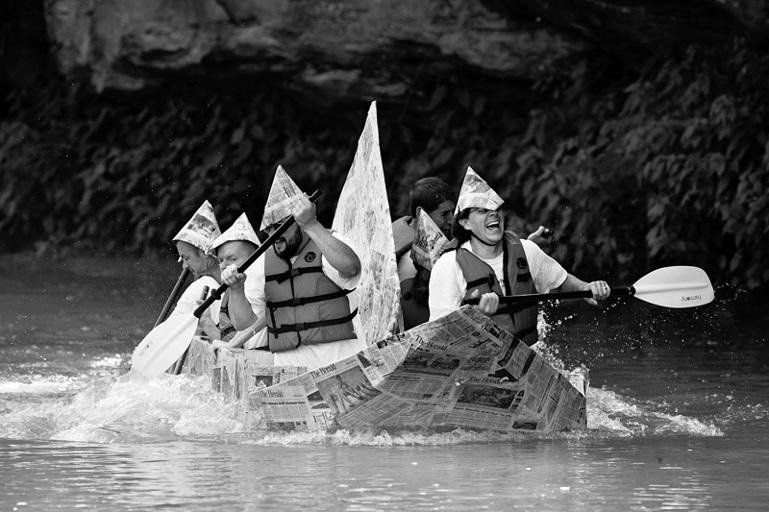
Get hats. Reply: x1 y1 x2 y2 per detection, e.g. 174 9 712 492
410 209 449 270
207 213 262 252
260 165 309 231
451 167 505 238
173 200 221 259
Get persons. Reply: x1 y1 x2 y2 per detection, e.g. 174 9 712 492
220 165 362 372
194 211 271 353
167 200 223 321
396 178 554 329
429 165 611 350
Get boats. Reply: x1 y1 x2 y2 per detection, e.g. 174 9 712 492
174 302 589 440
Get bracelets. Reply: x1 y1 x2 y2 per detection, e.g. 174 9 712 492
250 327 255 335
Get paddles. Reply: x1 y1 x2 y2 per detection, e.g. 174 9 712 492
132 189 322 373
461 265 714 308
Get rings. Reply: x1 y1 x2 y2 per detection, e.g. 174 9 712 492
603 286 608 289
